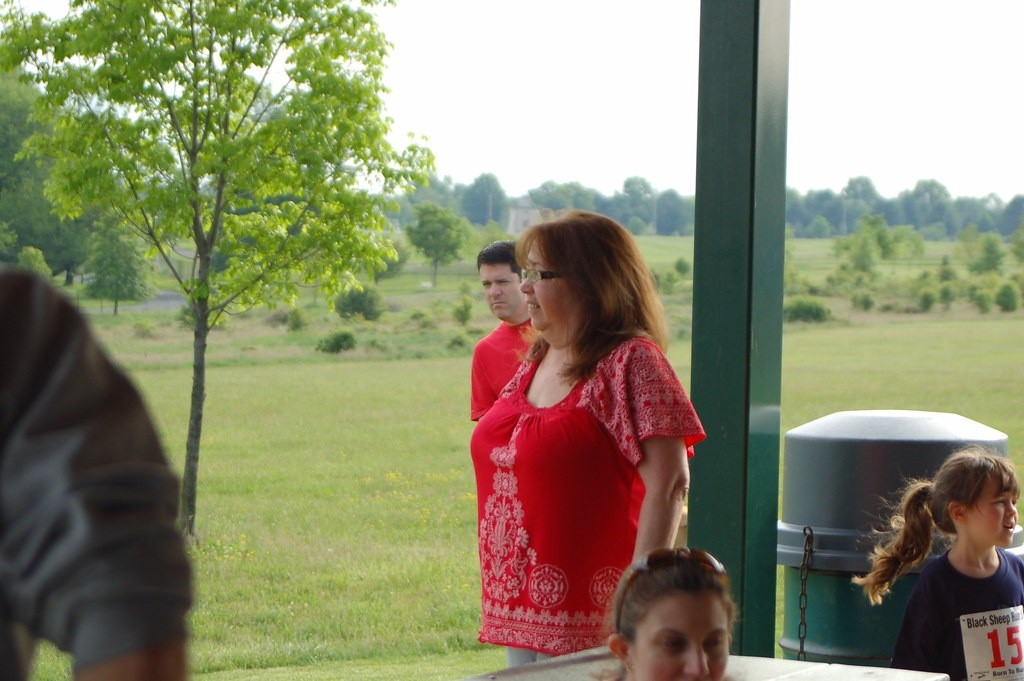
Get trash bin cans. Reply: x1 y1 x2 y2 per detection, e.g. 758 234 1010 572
776 409 1024 668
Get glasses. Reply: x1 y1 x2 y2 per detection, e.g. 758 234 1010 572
615 545 728 634
520 266 562 284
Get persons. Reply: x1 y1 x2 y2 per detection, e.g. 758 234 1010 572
851 451 1023 681
471 240 541 423
607 546 736 681
471 214 708 670
0 268 193 681
